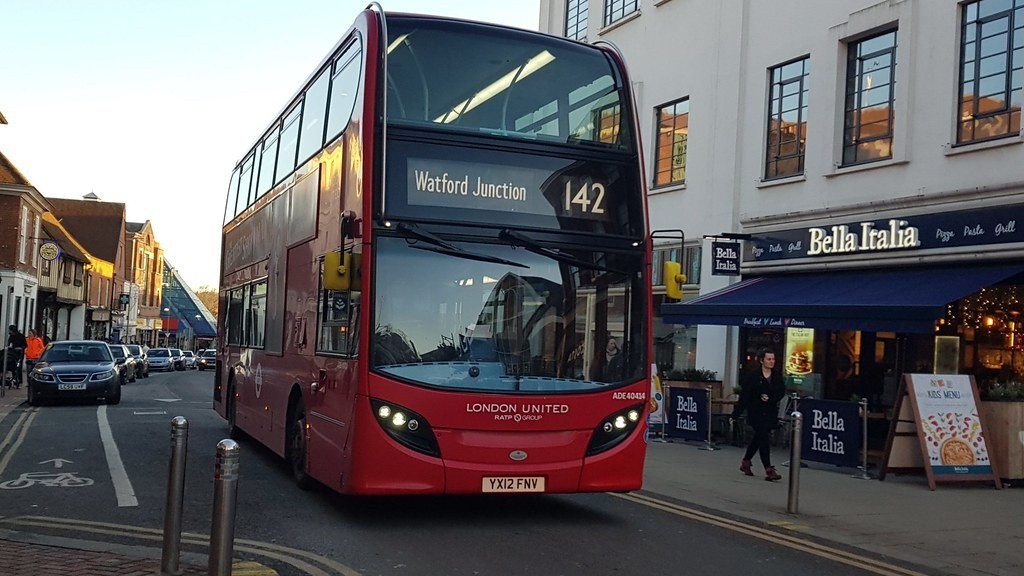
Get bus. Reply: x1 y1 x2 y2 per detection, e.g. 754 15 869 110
213 2 652 494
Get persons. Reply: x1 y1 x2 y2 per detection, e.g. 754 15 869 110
729 349 786 481
604 337 621 366
8 325 44 388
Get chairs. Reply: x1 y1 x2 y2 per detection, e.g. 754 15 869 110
89 348 104 360
52 350 68 360
770 395 789 448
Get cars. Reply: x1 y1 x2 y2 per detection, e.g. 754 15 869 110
182 351 196 370
125 345 148 379
169 349 186 371
145 348 175 372
27 340 121 406
197 349 216 371
195 349 206 362
107 345 136 385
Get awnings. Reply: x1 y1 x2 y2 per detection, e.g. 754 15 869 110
661 264 1024 333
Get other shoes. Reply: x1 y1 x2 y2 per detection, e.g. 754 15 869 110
740 460 754 476
765 466 782 481
